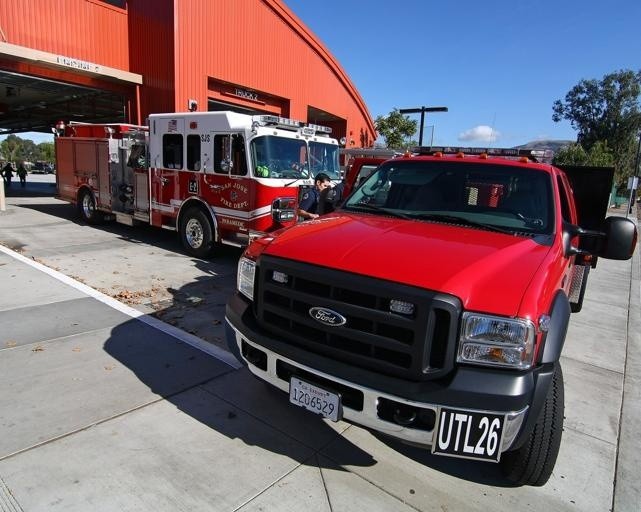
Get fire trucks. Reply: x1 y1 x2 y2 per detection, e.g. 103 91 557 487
51 110 343 258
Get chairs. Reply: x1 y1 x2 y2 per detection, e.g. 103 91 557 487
404 182 448 212
497 191 537 218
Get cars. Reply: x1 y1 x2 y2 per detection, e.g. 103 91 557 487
31 160 50 174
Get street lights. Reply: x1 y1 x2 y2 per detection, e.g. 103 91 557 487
400 106 448 146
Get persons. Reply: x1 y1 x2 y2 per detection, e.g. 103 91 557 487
14 163 27 188
0 162 16 186
296 172 332 221
